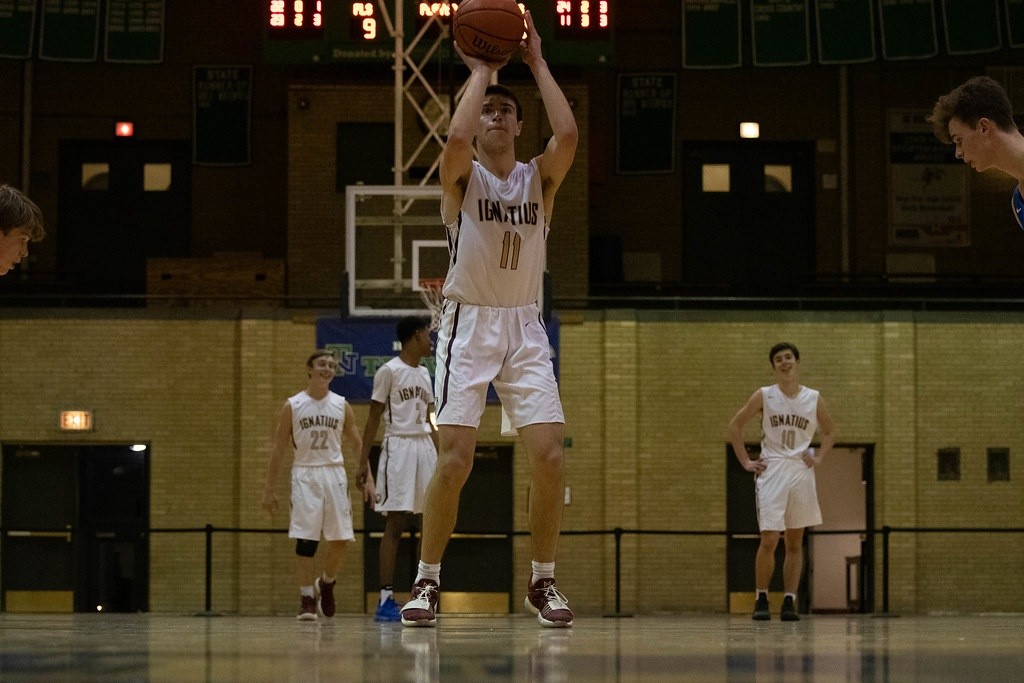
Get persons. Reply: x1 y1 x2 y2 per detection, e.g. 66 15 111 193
923 74 1024 232
727 339 835 624
353 316 443 624
257 349 378 625
0 183 47 280
398 8 583 630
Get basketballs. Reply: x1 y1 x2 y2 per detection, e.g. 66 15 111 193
453 1 524 61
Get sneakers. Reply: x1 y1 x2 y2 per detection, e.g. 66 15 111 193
297 595 318 621
315 577 337 618
525 571 575 627
398 578 440 626
375 595 401 621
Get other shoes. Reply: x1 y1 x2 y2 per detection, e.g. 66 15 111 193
752 592 772 620
780 595 801 621
